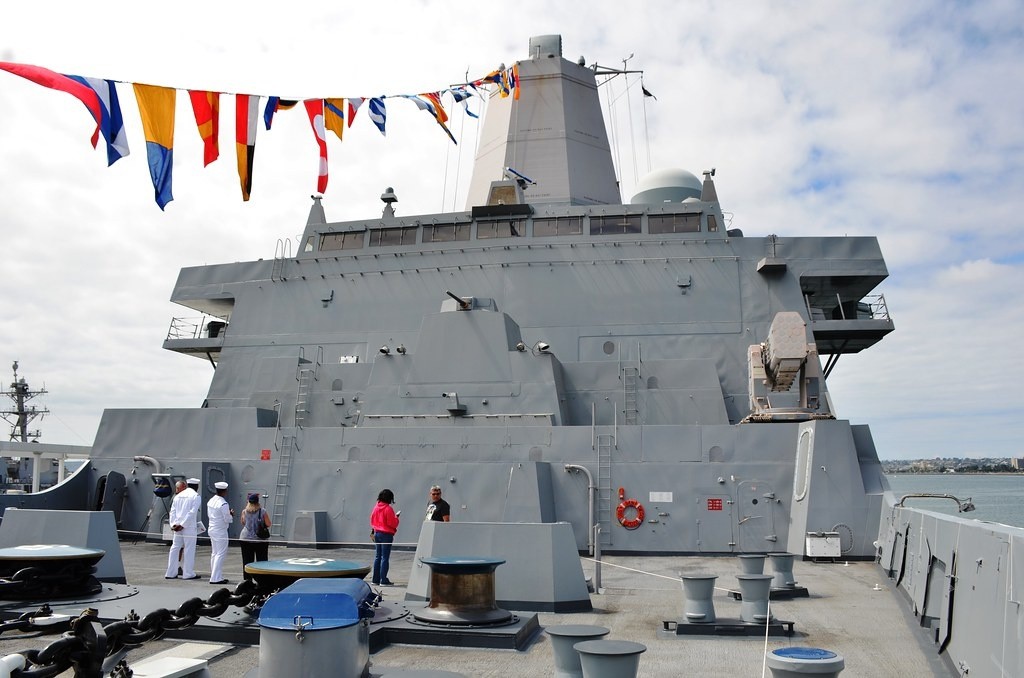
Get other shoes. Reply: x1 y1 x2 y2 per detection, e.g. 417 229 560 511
210 578 229 584
380 582 394 586
186 575 201 579
165 575 178 579
178 566 183 575
371 582 379 586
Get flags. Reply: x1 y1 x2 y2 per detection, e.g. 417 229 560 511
264 65 519 193
187 89 220 167
0 62 101 150
132 83 176 211
64 75 131 167
236 95 260 201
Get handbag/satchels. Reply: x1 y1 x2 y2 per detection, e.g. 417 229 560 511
255 508 270 539
370 528 374 542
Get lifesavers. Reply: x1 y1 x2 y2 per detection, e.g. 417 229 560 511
617 499 644 527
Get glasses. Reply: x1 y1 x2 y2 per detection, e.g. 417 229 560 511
432 493 439 496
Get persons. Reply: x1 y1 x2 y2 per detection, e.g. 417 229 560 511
207 482 234 584
170 481 186 576
165 478 202 580
425 486 450 522
240 493 271 580
370 489 399 586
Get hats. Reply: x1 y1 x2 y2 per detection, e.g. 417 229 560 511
249 493 259 504
187 478 200 484
214 482 228 489
